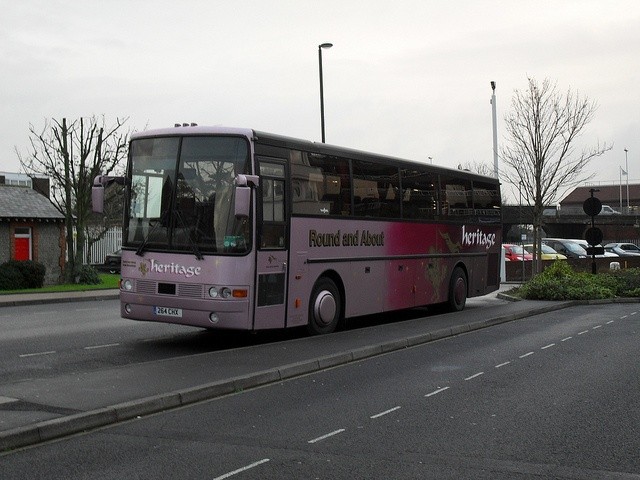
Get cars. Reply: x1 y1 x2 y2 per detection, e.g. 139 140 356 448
502 244 534 261
604 242 640 256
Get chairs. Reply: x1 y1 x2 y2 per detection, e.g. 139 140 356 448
321 192 362 214
360 197 400 216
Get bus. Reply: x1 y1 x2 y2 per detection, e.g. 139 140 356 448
119 122 502 335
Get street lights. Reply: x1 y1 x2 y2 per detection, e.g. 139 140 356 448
518 180 523 241
317 41 335 144
624 148 628 207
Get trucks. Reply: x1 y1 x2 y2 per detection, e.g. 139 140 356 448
542 238 619 258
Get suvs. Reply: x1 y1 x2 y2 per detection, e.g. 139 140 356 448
548 241 587 259
521 244 567 259
